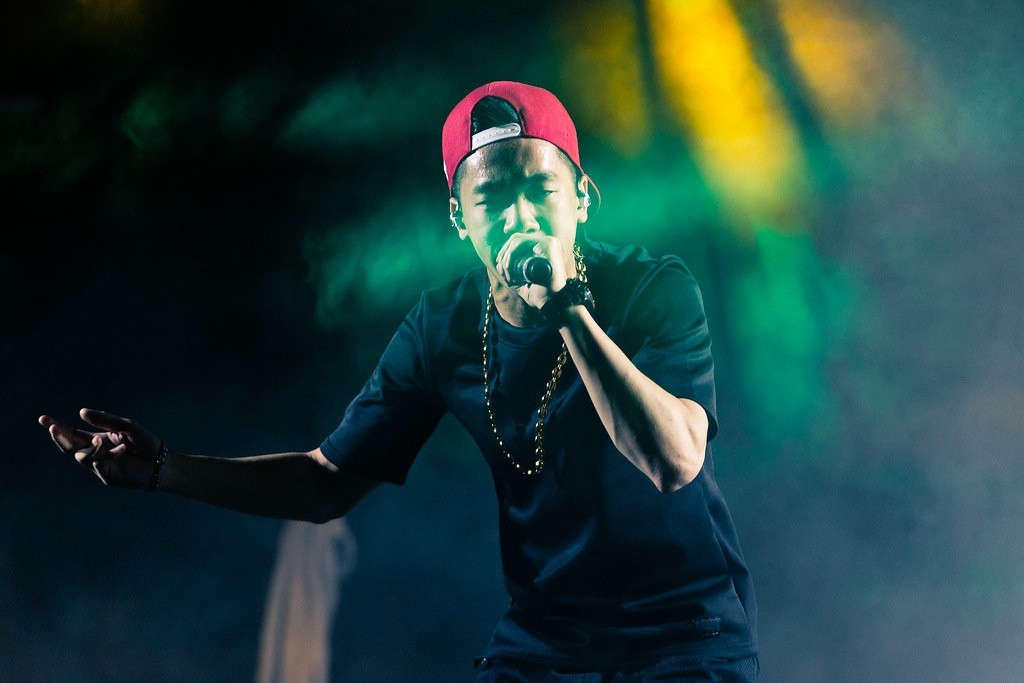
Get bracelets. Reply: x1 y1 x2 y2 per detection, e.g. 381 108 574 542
150 440 169 492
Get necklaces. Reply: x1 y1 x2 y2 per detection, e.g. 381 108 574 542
482 245 587 479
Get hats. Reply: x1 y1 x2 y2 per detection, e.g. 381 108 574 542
441 81 601 225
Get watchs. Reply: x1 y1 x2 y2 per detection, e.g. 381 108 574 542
540 277 597 322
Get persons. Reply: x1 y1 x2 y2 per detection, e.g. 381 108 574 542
38 80 762 683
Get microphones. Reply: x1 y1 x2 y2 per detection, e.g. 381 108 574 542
506 253 553 286
